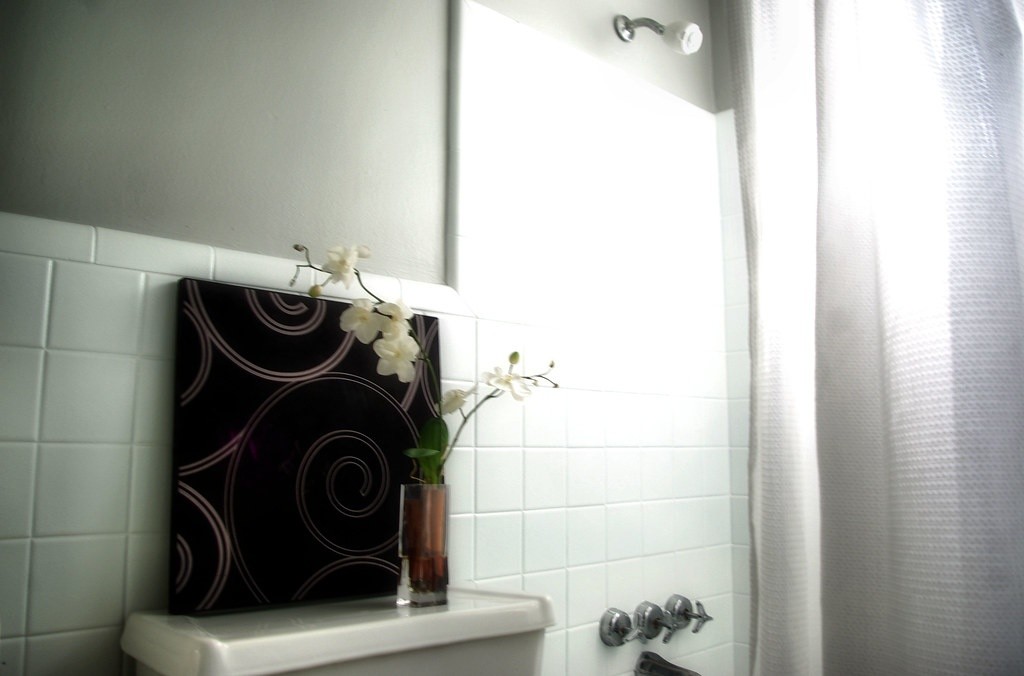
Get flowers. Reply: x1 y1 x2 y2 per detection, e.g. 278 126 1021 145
289 242 561 492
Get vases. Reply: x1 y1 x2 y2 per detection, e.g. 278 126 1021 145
397 484 449 609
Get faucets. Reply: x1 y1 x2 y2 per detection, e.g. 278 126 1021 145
632 651 701 676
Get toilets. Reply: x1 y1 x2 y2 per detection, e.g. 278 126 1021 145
120 587 558 676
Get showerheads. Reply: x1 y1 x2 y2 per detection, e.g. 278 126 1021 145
662 19 704 56
